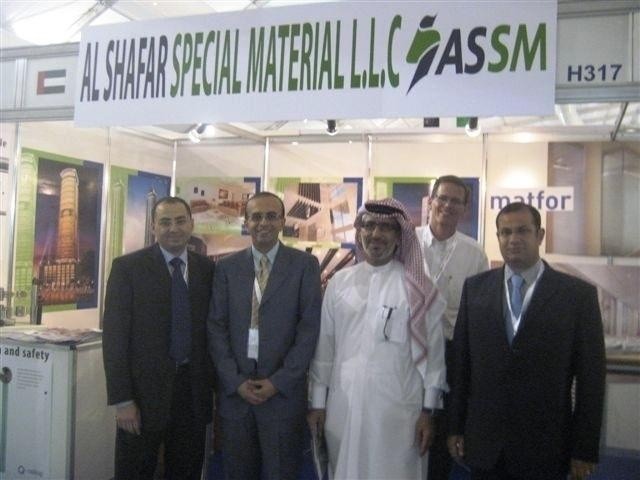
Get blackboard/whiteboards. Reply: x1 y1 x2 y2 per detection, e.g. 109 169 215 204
0 337 78 480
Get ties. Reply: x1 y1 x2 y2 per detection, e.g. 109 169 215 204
251 255 269 328
511 274 525 320
169 258 192 362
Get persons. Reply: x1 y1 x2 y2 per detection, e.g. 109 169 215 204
204 190 322 480
304 195 449 479
102 196 215 479
440 200 606 480
412 175 489 478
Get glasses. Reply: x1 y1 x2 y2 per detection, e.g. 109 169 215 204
362 221 399 233
434 192 464 207
247 212 282 221
